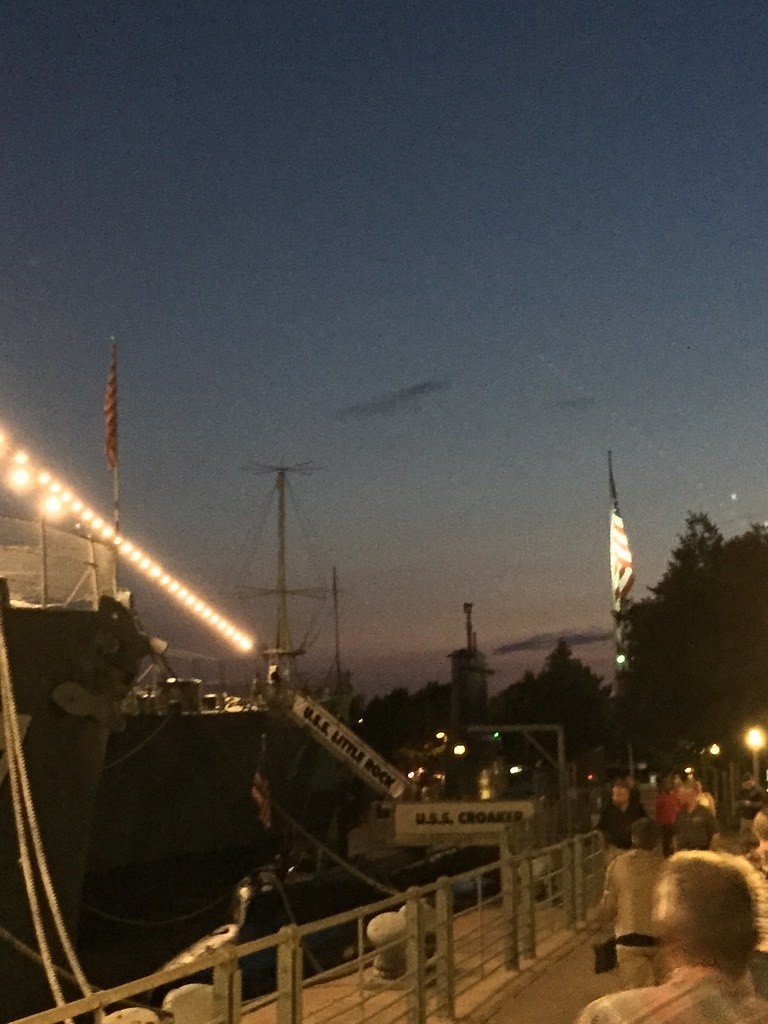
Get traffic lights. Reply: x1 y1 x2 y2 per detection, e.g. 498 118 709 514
492 732 501 741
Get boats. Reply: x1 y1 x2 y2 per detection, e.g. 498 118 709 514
91 677 358 949
0 515 166 1024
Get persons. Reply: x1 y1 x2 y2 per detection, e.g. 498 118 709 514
249 664 284 701
575 768 768 1024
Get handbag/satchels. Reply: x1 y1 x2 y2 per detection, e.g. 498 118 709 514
592 937 617 974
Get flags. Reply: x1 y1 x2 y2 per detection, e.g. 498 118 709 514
607 450 635 623
104 337 118 474
252 733 271 828
